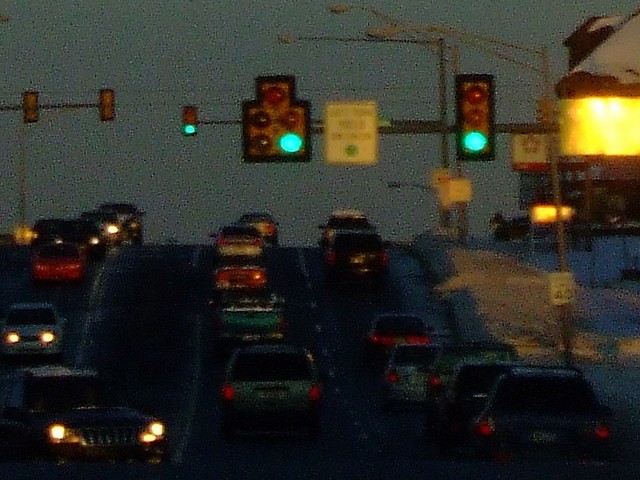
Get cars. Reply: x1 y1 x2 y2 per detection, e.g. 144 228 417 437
208 225 265 264
22 235 88 283
218 342 322 437
328 231 389 280
98 203 143 247
82 211 121 245
363 313 435 348
473 368 611 464
207 294 288 345
33 404 168 463
243 214 281 246
320 216 377 248
39 220 106 264
381 344 447 411
1 300 65 355
445 352 532 448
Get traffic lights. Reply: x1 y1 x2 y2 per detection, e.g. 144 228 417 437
246 76 305 155
461 75 489 153
182 104 196 134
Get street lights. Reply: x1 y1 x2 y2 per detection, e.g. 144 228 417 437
386 180 449 231
278 33 453 229
22 91 39 124
363 22 574 367
100 89 115 122
